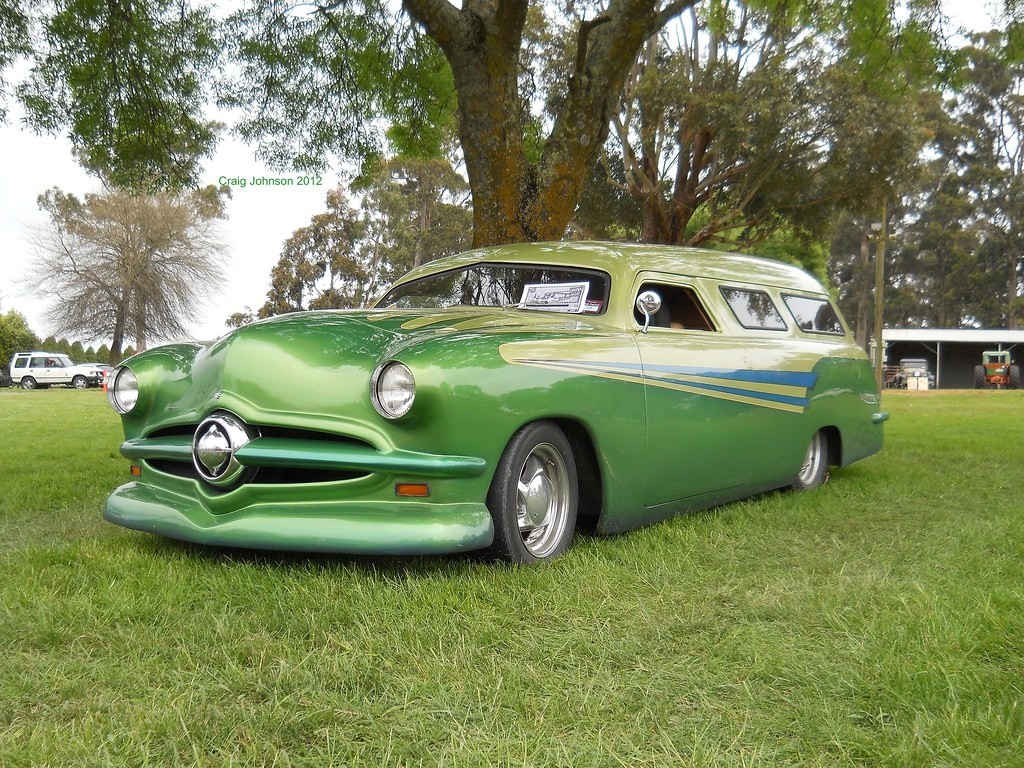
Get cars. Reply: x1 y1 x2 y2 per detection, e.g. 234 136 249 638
79 363 114 384
103 241 890 570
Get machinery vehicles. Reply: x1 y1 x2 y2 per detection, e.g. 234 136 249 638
974 350 1021 389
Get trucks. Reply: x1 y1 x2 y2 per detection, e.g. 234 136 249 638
886 357 935 390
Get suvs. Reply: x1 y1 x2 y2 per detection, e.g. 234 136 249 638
7 351 103 390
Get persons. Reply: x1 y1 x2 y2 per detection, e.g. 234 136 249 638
51 361 56 366
